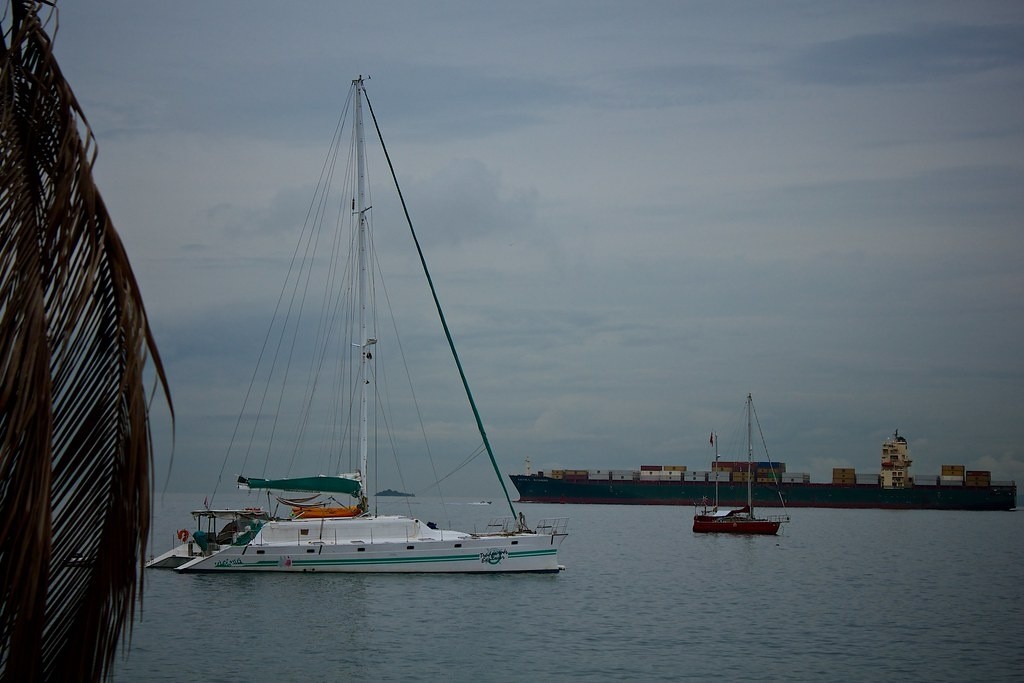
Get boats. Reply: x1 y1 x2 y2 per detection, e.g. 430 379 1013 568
508 426 1018 512
693 392 792 534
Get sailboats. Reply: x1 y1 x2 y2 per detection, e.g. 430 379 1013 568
147 75 573 574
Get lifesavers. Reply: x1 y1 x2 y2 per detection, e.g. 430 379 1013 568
177 529 188 542
